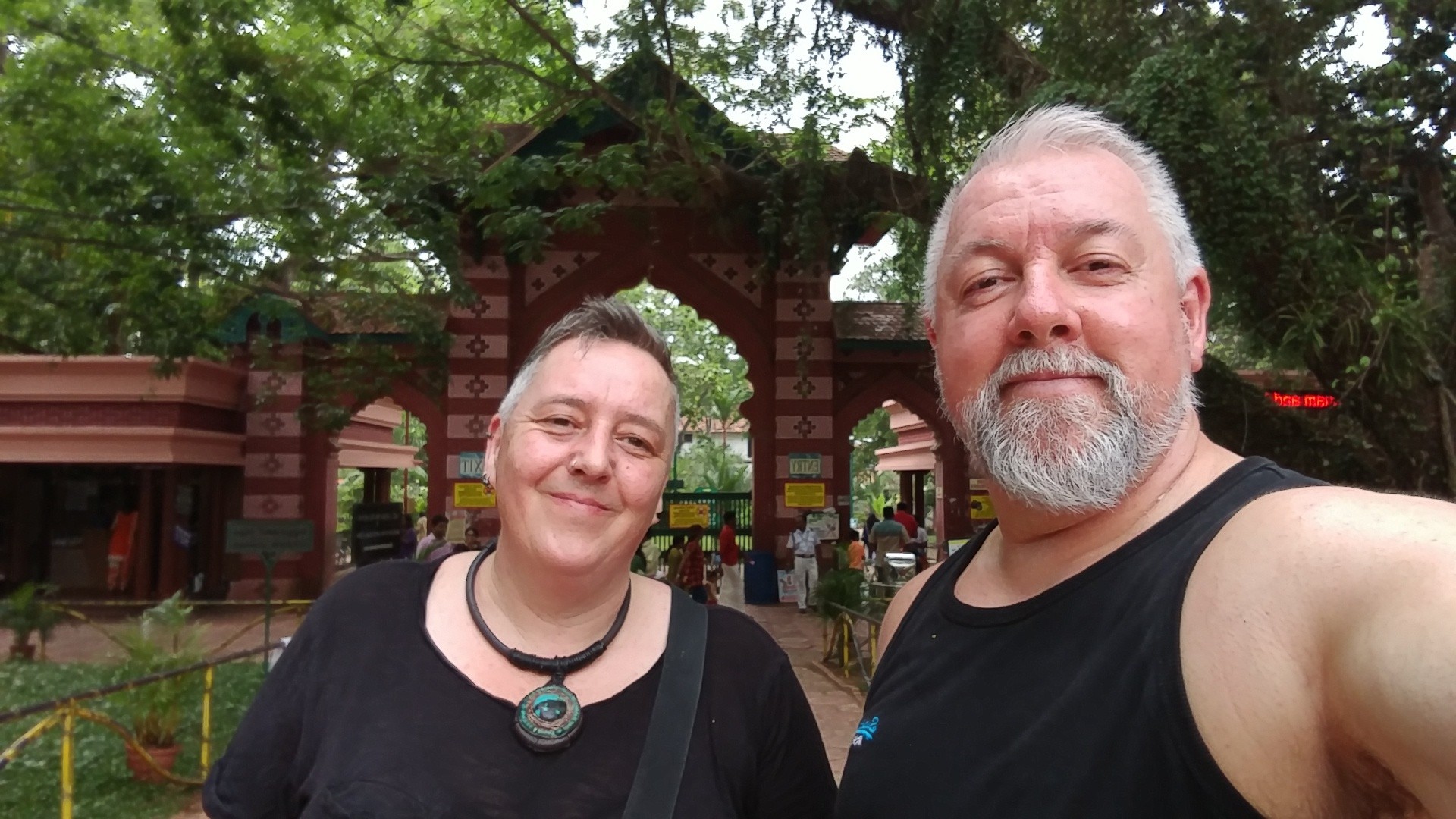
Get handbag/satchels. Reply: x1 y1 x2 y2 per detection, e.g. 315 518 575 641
784 531 795 573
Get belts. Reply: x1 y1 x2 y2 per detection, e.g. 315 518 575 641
795 553 816 559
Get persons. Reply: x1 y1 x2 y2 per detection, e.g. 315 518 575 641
846 531 869 575
862 513 880 559
414 512 427 539
894 502 928 555
416 514 448 560
465 526 479 546
630 527 661 577
833 103 1456 819
663 511 745 611
786 515 820 613
200 299 838 819
107 495 139 596
868 506 918 582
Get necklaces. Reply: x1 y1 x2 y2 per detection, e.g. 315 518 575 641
466 540 632 752
